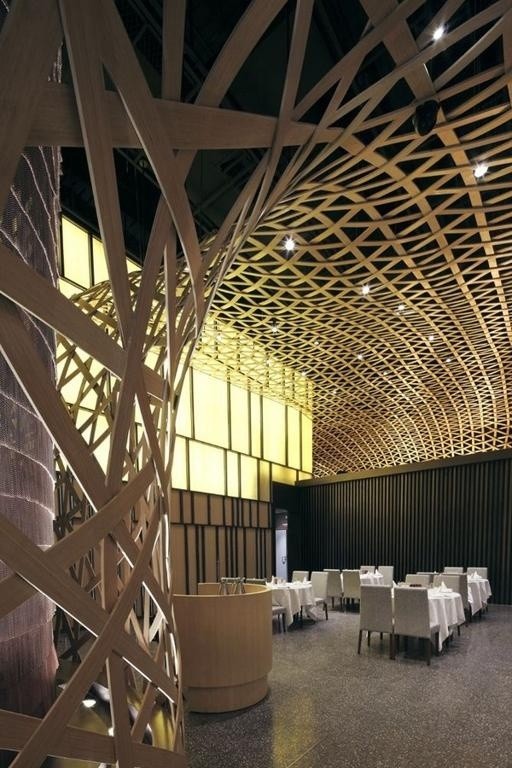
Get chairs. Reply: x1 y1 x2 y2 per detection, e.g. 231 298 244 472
358 584 400 660
291 571 309 619
377 566 393 588
341 570 361 613
406 574 430 586
360 566 375 573
304 571 329 621
433 575 461 640
467 567 488 612
324 568 343 608
438 572 473 628
444 567 463 573
272 607 286 634
